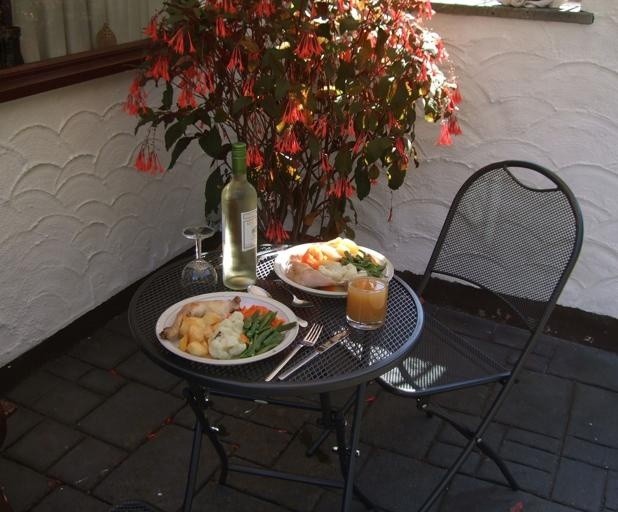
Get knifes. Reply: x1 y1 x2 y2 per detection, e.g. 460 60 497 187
276 329 353 380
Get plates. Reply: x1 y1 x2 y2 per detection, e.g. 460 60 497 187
153 291 298 367
273 242 394 299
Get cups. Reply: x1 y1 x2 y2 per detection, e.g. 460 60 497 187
346 275 390 331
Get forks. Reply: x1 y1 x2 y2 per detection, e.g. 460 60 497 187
263 320 324 382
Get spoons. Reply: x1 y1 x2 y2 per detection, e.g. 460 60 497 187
243 282 306 330
273 278 315 309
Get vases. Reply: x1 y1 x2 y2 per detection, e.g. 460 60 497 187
260 230 320 246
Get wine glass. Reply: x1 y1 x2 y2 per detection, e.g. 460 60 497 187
179 226 219 296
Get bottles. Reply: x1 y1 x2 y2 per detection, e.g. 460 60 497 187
221 140 258 290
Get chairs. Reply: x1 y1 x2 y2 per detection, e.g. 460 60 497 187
304 157 589 512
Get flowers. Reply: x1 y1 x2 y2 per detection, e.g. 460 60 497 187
126 1 469 247
